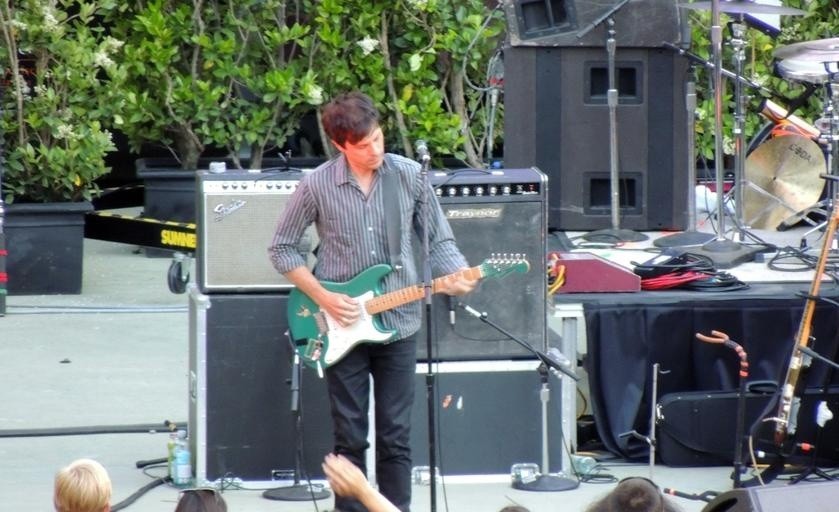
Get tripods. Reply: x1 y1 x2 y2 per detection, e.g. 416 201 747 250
702 72 839 282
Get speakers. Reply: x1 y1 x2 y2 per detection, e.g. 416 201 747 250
502 0 691 47
502 47 694 233
698 481 839 512
196 170 320 293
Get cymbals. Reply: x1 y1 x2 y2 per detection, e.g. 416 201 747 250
676 0 802 16
738 135 828 231
767 36 838 78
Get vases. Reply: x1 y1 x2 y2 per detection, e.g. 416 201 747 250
2 201 95 297
135 157 326 227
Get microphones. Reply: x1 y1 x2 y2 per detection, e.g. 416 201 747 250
575 0 630 39
415 139 430 162
446 292 456 325
660 41 715 69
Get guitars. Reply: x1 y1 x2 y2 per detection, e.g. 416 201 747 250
287 254 530 381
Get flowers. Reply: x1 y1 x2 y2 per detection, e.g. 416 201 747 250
1 0 125 201
95 0 505 171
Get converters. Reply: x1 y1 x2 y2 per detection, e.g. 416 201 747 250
410 167 549 361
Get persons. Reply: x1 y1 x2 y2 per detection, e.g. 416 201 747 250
496 505 530 512
322 452 406 512
588 477 680 512
175 488 227 512
52 457 113 512
269 92 478 512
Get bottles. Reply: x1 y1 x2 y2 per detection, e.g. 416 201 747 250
169 431 191 486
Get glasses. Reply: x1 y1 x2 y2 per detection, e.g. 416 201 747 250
179 488 217 503
